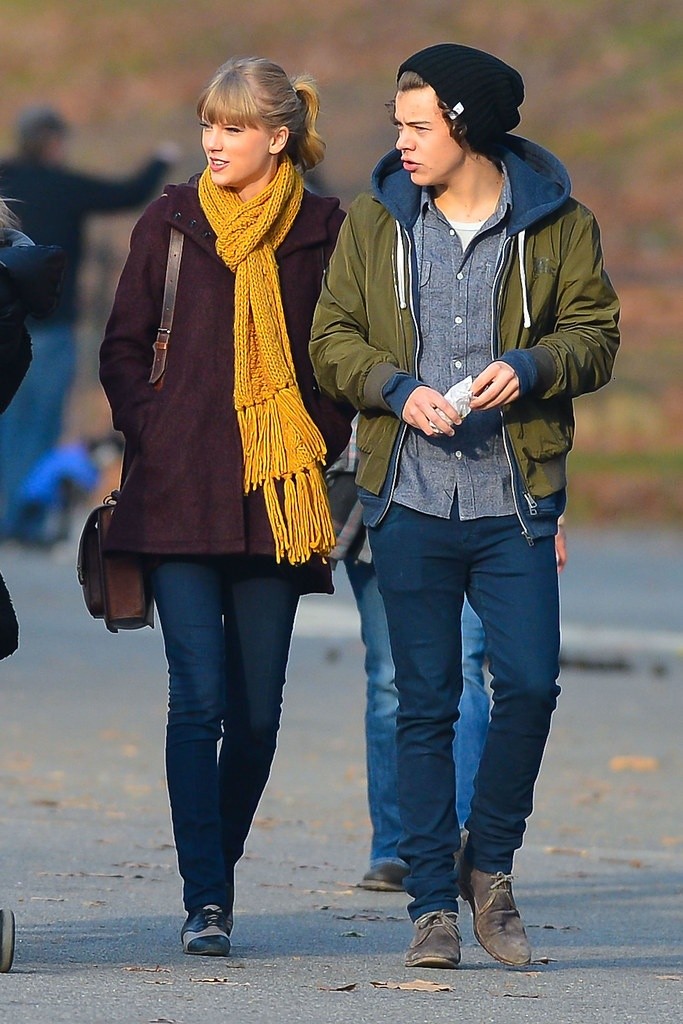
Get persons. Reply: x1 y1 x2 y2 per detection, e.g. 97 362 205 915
0 45 620 973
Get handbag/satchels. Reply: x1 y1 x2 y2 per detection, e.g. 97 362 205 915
76 490 154 633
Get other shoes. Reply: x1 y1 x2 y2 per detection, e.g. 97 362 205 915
360 863 409 891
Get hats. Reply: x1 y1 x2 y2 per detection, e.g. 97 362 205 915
397 43 526 147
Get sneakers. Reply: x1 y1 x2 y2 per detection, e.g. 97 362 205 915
454 851 532 966
180 904 233 956
408 907 464 966
